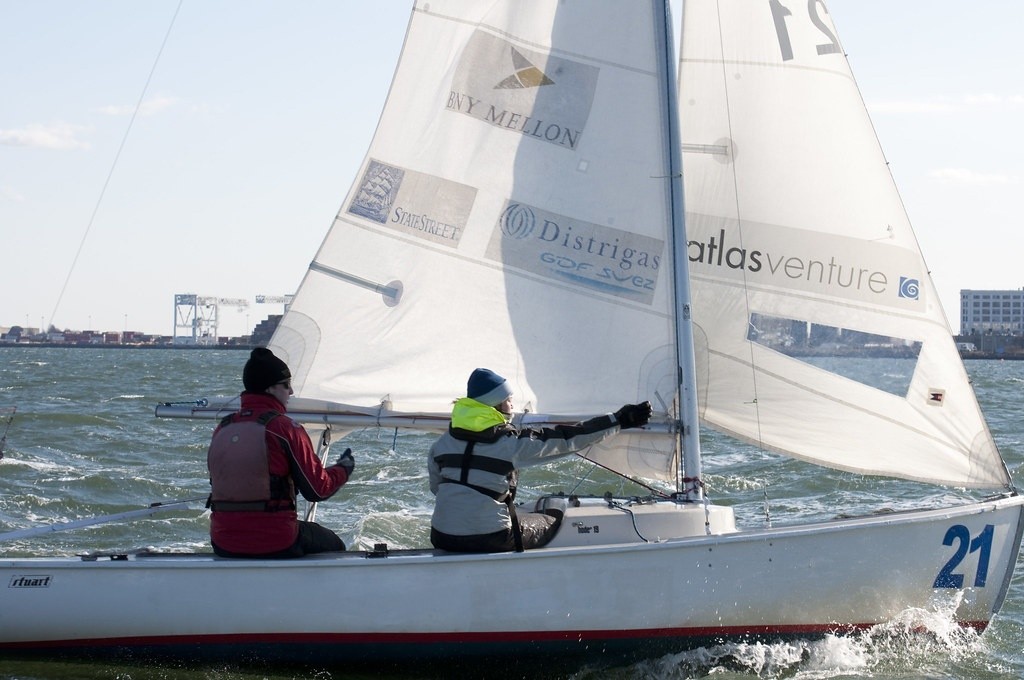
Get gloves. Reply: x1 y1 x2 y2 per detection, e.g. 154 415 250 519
336 447 354 476
613 401 653 428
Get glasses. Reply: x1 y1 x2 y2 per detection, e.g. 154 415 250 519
274 379 291 390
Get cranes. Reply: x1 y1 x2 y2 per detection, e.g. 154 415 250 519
194 294 295 344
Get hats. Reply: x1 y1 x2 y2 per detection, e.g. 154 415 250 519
243 347 292 391
467 368 513 407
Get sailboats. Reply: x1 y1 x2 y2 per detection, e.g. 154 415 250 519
1 1 1024 656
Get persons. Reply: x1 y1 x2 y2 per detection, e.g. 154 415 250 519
207 348 355 560
428 367 653 551
